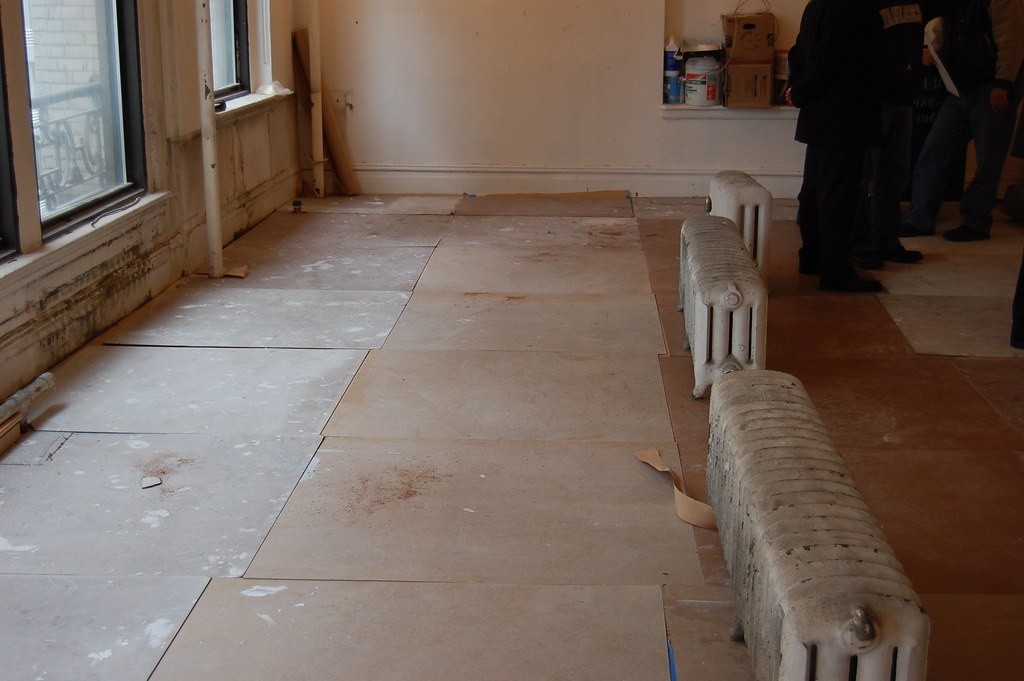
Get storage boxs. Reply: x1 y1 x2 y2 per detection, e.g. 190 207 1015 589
722 10 790 110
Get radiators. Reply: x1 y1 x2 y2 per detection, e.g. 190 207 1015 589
704 169 775 270
676 215 771 400
703 370 934 681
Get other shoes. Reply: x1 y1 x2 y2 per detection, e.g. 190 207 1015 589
845 243 884 269
818 262 883 292
872 237 923 262
798 248 866 274
942 224 991 241
896 223 936 237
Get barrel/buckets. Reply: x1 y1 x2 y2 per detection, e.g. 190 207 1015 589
685 57 720 106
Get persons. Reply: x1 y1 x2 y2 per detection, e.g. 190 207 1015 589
784 0 1024 349
